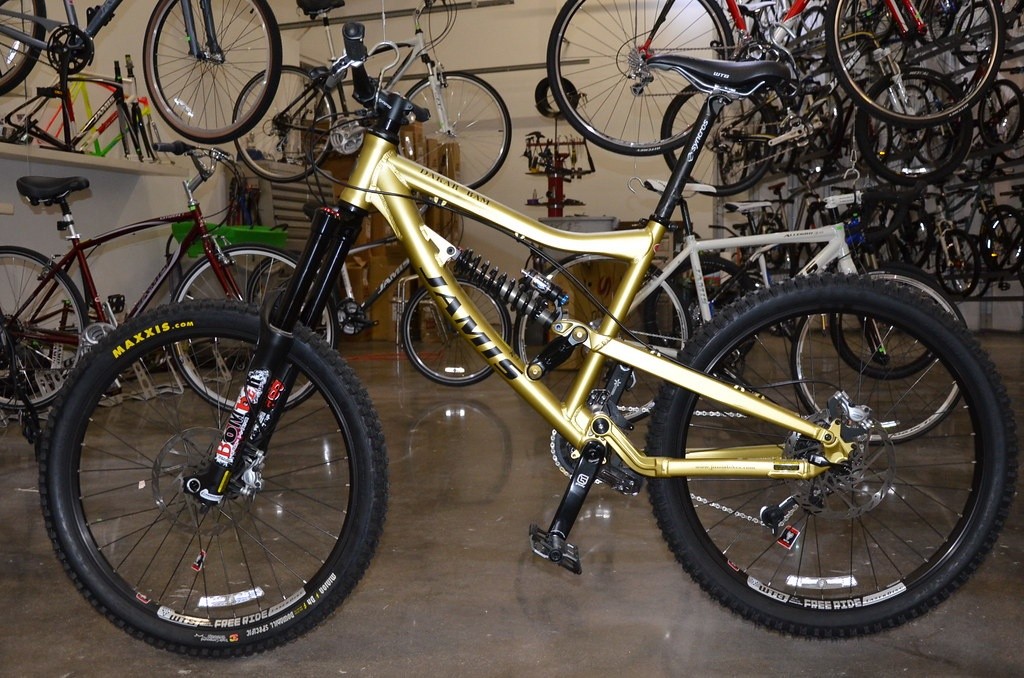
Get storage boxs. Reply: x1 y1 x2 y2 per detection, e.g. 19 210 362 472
301 118 458 345
539 231 675 370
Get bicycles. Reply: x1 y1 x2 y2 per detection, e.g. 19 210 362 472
0 0 1023 443
36 20 1017 660
